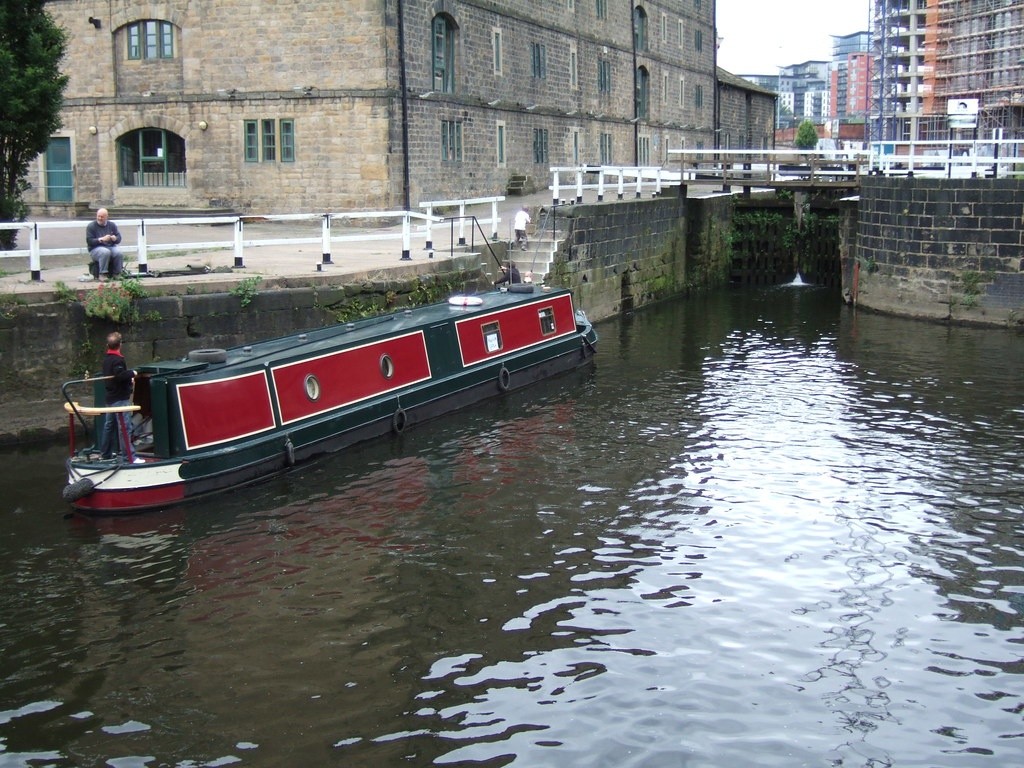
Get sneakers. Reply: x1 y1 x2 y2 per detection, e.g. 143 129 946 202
99 456 117 462
123 457 145 464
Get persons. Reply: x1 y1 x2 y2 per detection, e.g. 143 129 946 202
492 260 521 286
86 208 124 281
98 332 146 464
514 205 531 251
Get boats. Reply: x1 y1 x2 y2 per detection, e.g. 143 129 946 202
60 283 599 513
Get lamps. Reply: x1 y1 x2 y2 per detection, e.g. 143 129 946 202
89 126 98 134
199 120 208 130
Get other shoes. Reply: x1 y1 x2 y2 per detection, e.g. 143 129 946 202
109 273 124 281
522 247 527 251
99 273 109 282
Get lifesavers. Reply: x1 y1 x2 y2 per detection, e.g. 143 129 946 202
499 367 511 392
392 408 407 433
449 296 483 306
509 283 534 293
189 349 227 363
